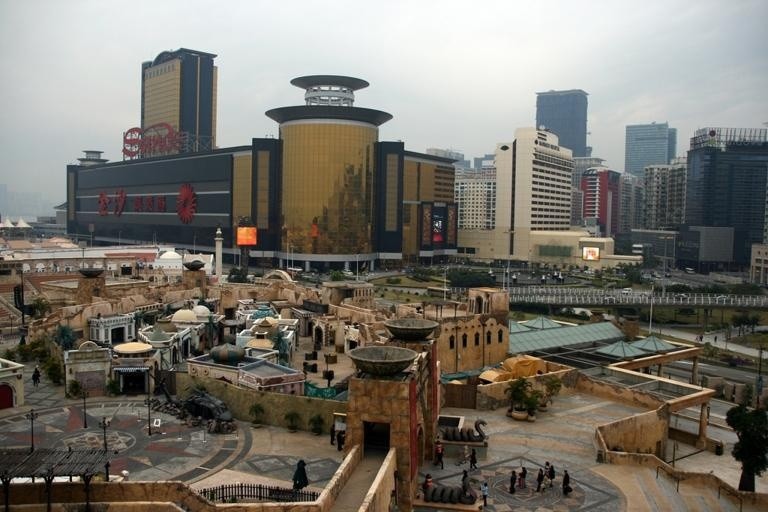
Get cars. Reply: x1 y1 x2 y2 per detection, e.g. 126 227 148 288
672 293 689 300
639 293 652 299
604 297 619 303
622 287 632 295
343 268 353 275
665 272 672 278
715 295 735 303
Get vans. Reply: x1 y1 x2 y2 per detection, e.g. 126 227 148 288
686 268 695 275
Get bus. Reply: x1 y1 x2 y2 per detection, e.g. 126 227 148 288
287 268 304 276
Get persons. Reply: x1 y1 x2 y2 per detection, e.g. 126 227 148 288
510 462 569 496
714 336 717 342
32 371 38 387
470 449 477 469
481 483 488 507
20 335 26 345
330 425 346 451
35 368 40 383
462 470 468 496
696 336 698 341
700 334 703 341
433 448 444 469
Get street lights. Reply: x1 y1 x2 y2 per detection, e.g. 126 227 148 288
503 229 517 288
659 235 673 298
25 409 39 450
8 314 14 335
98 416 111 450
356 253 362 280
649 281 656 333
290 245 295 279
442 267 449 301
79 391 90 428
755 346 767 404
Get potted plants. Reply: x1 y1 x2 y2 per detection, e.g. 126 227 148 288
44 363 63 387
307 413 324 435
502 368 563 422
105 381 118 397
67 379 83 400
283 411 302 432
248 402 266 428
